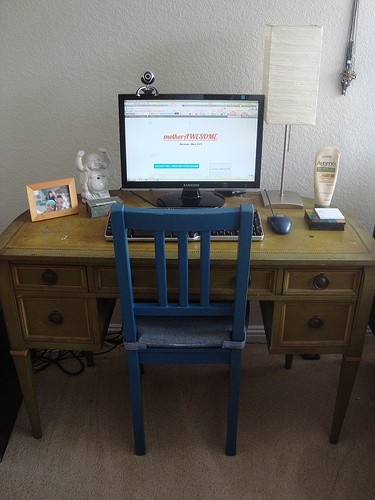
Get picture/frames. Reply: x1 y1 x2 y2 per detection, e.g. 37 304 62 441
25 177 80 222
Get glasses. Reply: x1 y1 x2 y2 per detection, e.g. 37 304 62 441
56 202 63 204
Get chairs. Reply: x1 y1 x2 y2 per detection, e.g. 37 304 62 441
109 204 254 455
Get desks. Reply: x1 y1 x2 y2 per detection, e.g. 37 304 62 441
0 191 375 445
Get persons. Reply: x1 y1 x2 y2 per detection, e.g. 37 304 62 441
42 190 67 213
75 148 111 199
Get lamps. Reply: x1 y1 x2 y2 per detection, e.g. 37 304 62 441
262 24 323 209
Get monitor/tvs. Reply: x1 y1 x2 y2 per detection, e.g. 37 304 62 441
117 93 265 208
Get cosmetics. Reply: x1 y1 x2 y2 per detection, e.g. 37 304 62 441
314 146 342 208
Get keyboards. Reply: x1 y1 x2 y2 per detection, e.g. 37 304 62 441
104 208 265 242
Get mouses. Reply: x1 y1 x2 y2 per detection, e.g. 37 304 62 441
267 214 291 234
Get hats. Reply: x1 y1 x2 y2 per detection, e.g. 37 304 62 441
45 200 56 206
